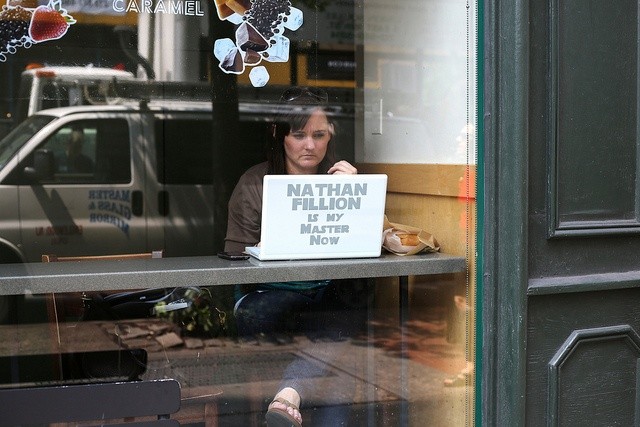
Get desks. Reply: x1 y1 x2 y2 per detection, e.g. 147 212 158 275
1 248 467 427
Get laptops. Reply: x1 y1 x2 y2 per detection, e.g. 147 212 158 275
246 174 388 261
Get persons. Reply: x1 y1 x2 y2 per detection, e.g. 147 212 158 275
225 90 357 426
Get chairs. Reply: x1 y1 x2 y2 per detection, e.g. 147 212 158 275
43 250 219 427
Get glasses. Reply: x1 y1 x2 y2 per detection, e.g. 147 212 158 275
272 86 329 138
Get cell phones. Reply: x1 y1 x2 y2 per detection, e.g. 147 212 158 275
216 252 250 261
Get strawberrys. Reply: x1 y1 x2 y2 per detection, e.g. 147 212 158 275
30 2 75 42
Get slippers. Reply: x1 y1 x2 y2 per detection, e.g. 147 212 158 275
265 397 302 427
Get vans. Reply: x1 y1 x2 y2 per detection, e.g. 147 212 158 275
0 98 354 324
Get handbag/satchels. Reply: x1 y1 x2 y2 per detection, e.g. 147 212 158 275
179 286 230 338
48 286 199 382
